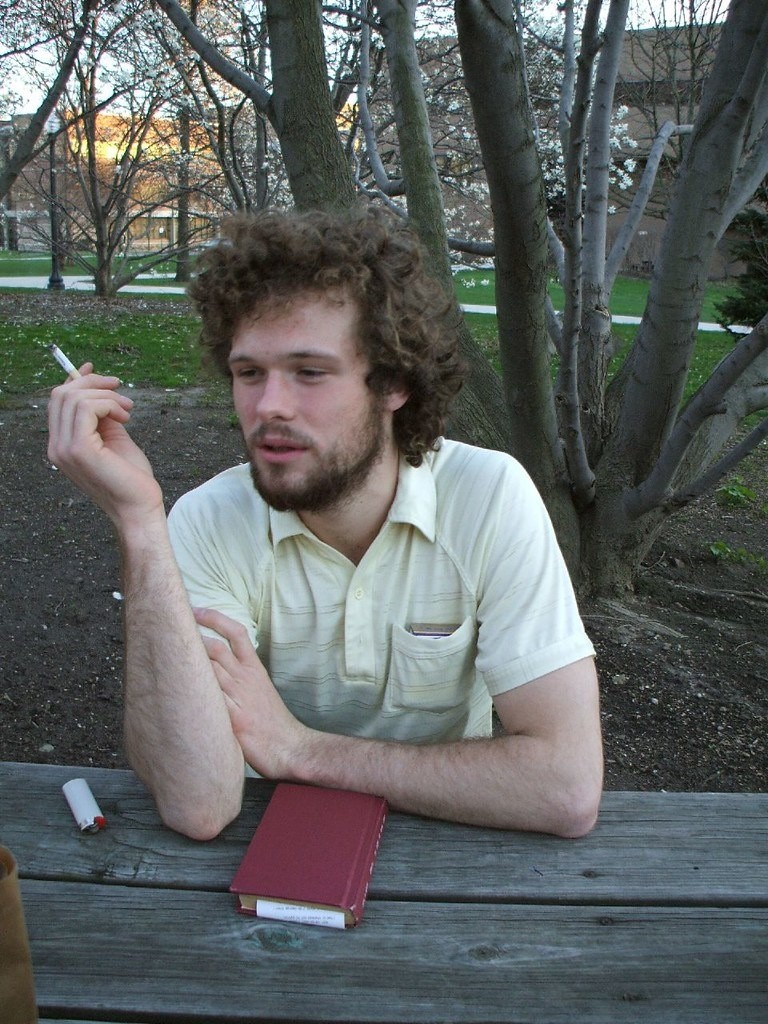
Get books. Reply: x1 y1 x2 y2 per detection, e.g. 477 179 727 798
229 782 390 929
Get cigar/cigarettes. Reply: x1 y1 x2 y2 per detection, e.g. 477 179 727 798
48 342 81 380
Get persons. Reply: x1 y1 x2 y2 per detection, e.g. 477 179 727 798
46 201 605 842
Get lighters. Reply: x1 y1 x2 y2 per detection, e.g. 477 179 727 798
61 778 105 834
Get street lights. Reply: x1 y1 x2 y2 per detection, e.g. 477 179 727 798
46 111 65 289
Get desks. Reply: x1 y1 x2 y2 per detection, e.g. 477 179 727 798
0 760 768 1024
